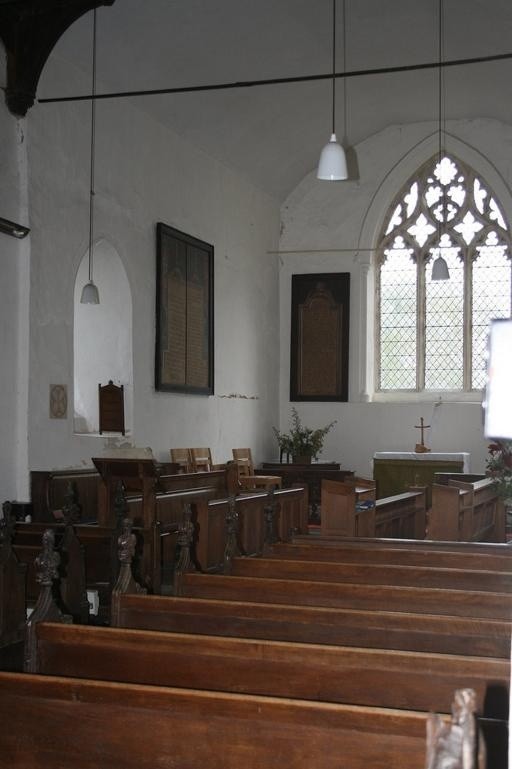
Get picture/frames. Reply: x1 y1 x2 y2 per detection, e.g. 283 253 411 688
292 274 349 400
154 223 213 394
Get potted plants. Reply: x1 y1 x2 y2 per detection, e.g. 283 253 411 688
271 406 337 464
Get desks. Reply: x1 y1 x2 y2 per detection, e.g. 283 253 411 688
372 451 469 504
261 461 340 504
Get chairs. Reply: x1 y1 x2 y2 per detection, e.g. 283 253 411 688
233 448 283 498
170 447 196 482
196 447 216 482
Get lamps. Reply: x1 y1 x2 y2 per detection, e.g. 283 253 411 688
78 0 101 306
430 0 451 281
316 0 348 182
